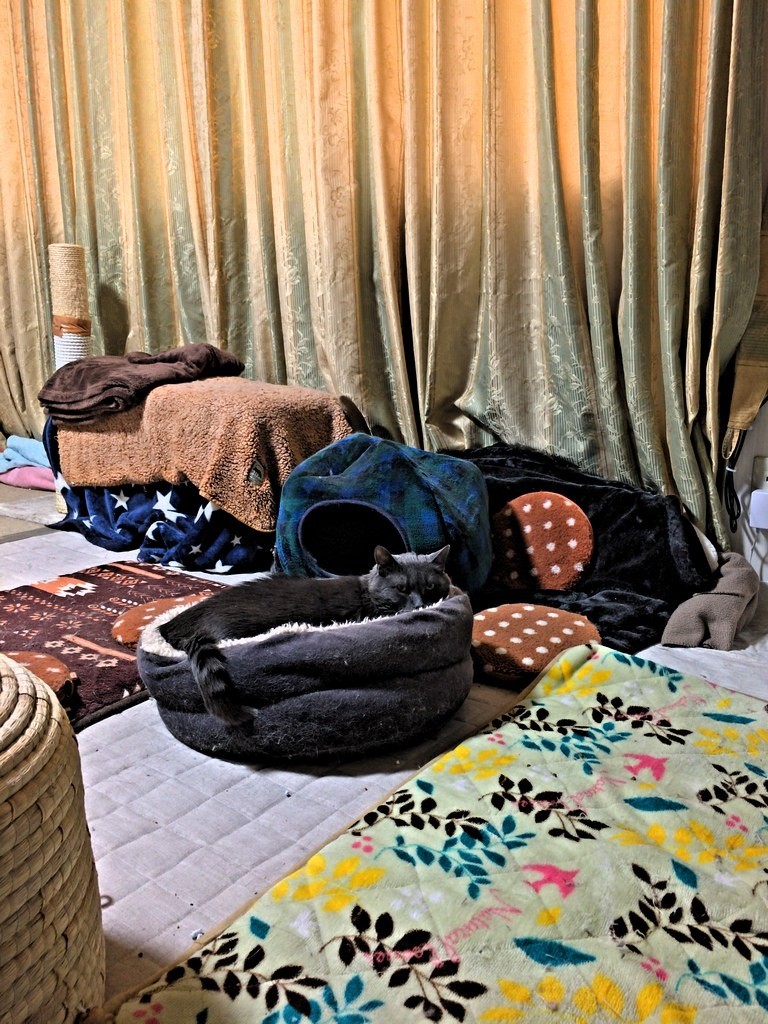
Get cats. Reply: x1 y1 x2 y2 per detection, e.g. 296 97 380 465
158 544 451 726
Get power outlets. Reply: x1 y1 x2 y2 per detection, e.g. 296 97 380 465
750 456 768 496
